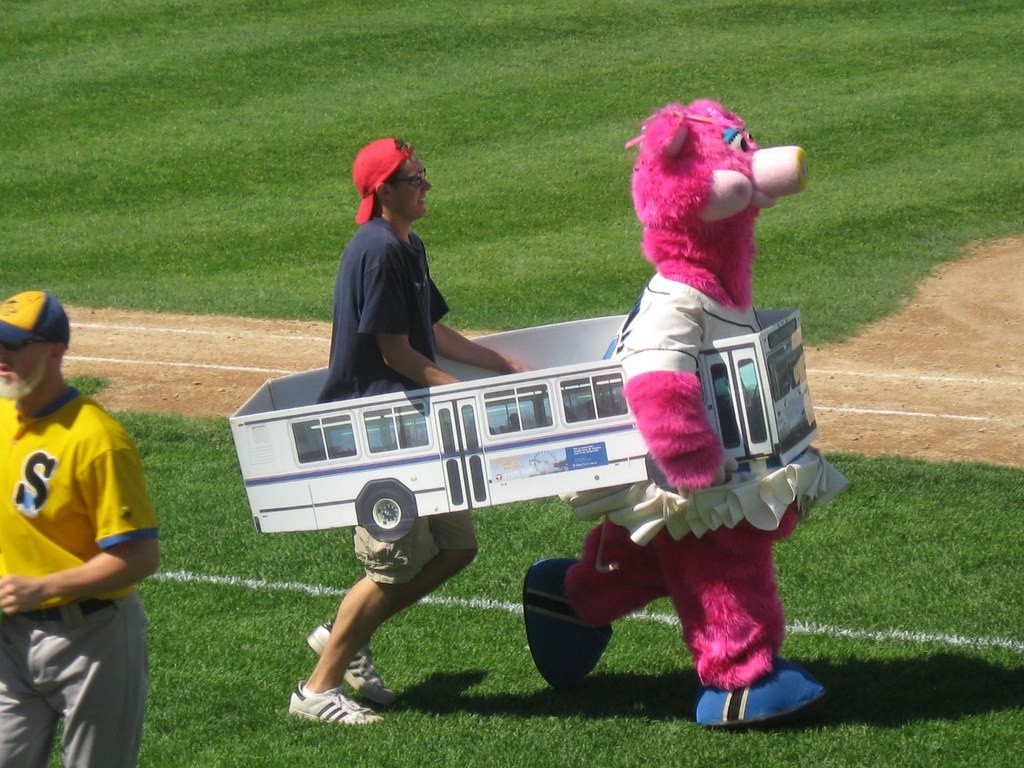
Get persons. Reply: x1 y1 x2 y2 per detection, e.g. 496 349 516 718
0 291 161 768
289 137 532 724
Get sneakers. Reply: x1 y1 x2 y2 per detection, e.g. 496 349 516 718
307 619 397 707
289 681 385 726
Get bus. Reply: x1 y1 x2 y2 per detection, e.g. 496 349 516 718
228 308 817 534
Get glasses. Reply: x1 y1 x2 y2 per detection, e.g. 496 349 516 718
398 167 426 186
0 338 49 351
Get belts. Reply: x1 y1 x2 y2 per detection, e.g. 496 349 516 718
21 598 113 621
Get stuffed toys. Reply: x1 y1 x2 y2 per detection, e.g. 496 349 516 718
523 97 853 728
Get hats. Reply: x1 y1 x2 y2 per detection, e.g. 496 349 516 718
0 290 69 344
353 137 412 224
